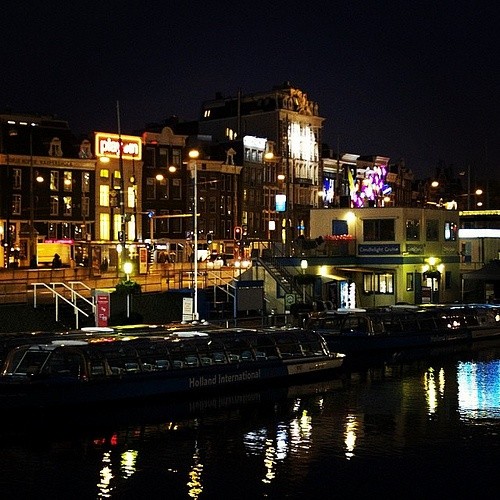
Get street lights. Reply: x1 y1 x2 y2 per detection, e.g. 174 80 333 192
188 149 199 321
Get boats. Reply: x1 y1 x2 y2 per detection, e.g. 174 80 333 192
299 301 500 358
0 323 347 416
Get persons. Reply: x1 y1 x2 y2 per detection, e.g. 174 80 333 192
353 166 390 206
76 253 89 265
53 253 63 268
93 257 109 268
446 223 457 240
